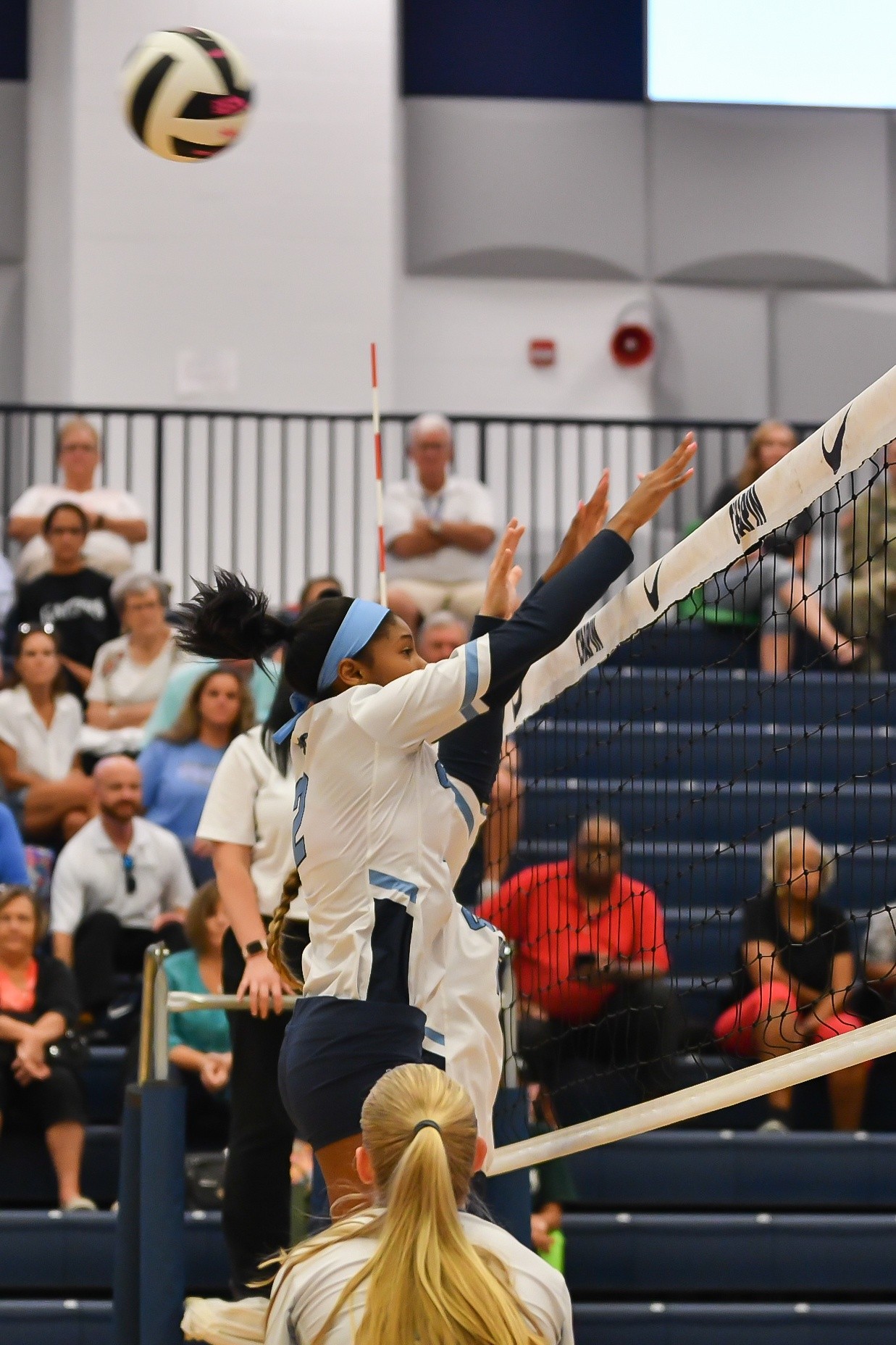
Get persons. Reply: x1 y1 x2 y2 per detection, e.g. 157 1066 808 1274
194 724 334 1300
1 421 896 1222
248 1062 574 1345
161 434 697 1223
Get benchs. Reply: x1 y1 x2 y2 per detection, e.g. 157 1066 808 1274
0 666 896 1345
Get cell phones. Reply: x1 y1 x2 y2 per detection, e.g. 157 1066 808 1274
574 953 596 973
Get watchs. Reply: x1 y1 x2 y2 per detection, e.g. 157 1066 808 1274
242 940 268 960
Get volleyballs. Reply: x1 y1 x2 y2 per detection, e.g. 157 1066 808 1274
119 26 251 162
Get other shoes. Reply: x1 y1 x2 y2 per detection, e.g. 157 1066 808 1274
758 1119 792 1135
66 1196 98 1215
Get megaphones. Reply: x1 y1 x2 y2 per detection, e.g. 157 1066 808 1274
608 324 659 369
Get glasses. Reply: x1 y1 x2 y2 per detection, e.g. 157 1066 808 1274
123 853 136 895
